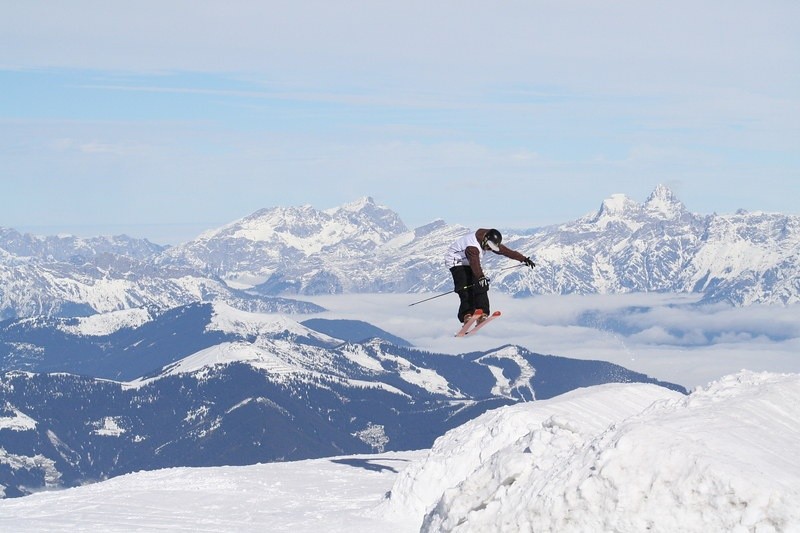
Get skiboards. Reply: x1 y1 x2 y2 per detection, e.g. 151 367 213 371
454 309 502 338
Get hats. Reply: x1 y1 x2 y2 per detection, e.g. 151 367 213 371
485 237 500 252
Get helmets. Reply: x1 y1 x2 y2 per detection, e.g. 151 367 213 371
485 229 502 245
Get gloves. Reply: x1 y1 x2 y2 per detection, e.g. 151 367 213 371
478 277 488 287
524 257 536 269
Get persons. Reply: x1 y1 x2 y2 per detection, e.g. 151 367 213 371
445 228 536 327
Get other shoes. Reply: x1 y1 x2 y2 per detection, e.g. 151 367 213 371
476 314 491 325
465 313 472 325
